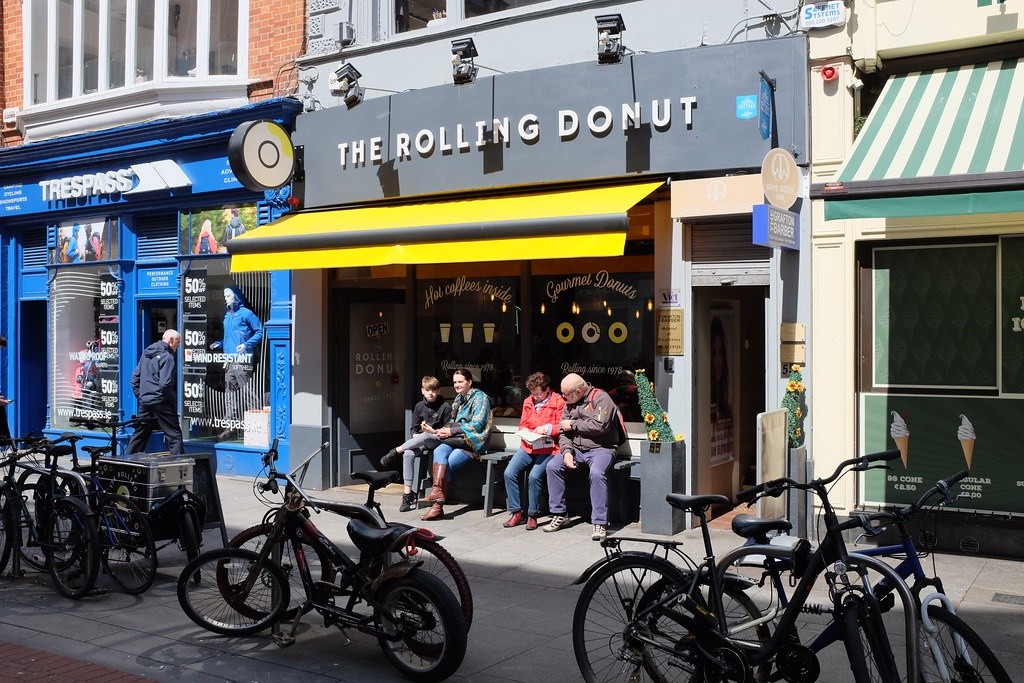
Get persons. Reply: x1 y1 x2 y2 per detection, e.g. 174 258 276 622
380 376 452 512
126 329 185 455
194 220 217 253
609 369 644 422
210 286 263 441
69 348 100 428
63 223 84 263
417 369 493 520
222 208 246 243
543 373 619 540
502 371 567 530
84 223 101 261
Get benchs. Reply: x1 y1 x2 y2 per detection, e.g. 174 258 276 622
414 449 516 518
525 460 638 523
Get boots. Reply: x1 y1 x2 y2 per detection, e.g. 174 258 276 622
421 479 448 521
417 462 445 504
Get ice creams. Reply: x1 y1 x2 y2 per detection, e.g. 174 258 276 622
890 411 910 468
958 414 976 470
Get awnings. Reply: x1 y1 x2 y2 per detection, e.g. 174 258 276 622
809 58 1024 221
226 182 666 274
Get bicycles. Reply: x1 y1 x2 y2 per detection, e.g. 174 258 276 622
0 417 204 600
174 439 468 683
214 442 475 659
570 449 901 683
707 472 1014 682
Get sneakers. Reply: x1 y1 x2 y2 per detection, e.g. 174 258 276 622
592 524 606 540
543 513 572 532
380 448 399 466
399 490 414 511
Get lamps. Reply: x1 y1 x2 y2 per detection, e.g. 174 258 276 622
595 14 635 66
330 63 401 111
451 37 506 86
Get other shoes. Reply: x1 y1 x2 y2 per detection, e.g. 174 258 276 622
218 427 236 441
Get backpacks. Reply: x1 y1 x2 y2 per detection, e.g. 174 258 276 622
199 237 211 254
590 388 627 446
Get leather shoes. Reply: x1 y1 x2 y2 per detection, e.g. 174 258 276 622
503 512 525 526
526 516 537 529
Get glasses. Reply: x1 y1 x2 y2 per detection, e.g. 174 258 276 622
530 391 543 397
562 390 576 397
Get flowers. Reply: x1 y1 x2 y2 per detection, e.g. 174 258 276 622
634 369 684 443
780 364 806 449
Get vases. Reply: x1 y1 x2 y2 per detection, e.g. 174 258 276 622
640 440 686 536
788 444 807 539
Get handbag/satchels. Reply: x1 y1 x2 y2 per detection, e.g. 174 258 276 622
440 418 469 447
